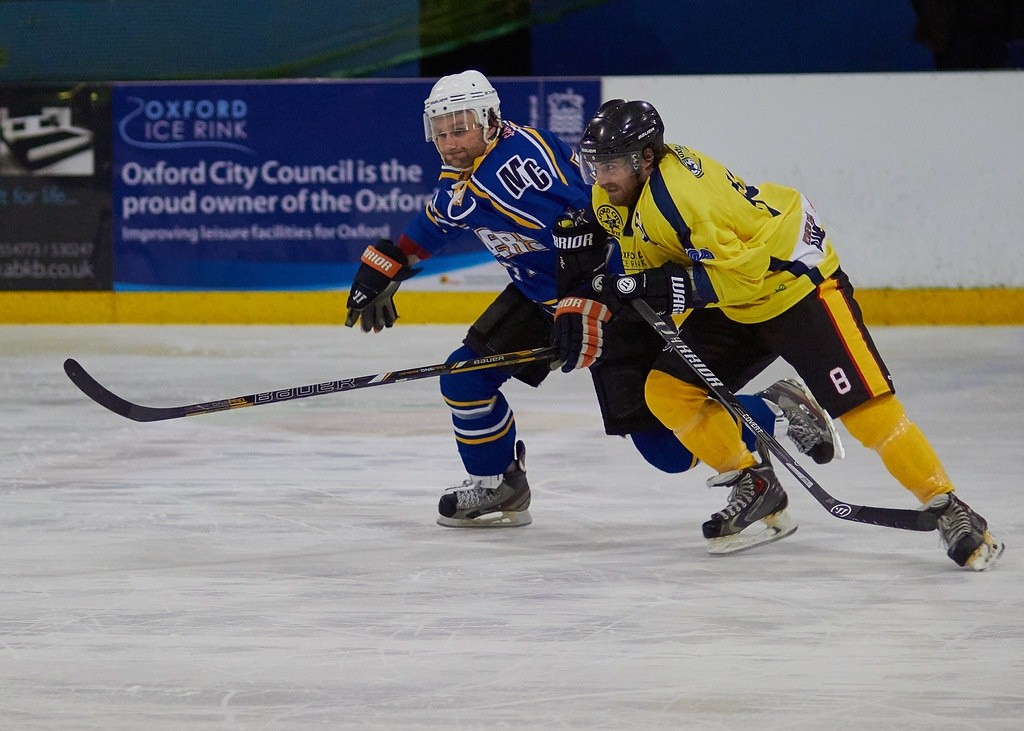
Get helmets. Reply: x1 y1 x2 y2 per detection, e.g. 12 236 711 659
579 99 665 175
423 70 501 142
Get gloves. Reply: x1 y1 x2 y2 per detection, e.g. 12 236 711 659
548 296 613 373
344 239 424 333
551 216 609 301
601 260 691 324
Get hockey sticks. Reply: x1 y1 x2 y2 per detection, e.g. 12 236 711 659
630 296 940 533
63 344 565 423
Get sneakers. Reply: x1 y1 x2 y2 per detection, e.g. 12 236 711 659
754 377 846 465
436 440 533 528
702 439 799 555
936 492 1006 571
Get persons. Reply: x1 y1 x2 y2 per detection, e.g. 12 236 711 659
344 69 845 530
577 98 1005 572
